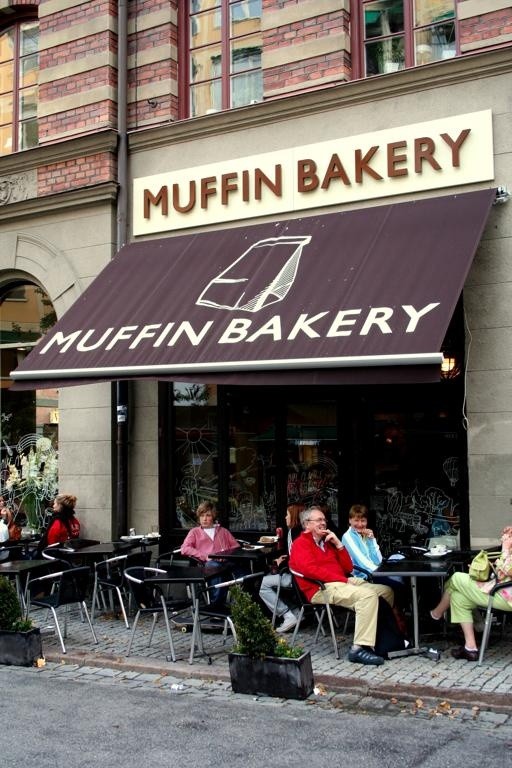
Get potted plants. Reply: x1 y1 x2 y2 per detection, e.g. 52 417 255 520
422 9 457 59
221 580 321 702
1 572 42 669
373 39 408 74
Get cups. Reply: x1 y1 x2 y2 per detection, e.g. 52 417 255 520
20 526 40 538
129 528 136 539
150 525 158 538
435 545 446 552
430 548 439 554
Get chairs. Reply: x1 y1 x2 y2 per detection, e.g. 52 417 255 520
123 537 512 664
1 537 153 654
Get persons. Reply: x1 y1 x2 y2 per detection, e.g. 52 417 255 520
419 495 511 661
180 500 243 620
288 505 398 666
0 492 23 542
32 495 82 599
340 502 412 611
256 502 311 634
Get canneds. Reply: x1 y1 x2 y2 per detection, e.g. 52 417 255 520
276 526 284 540
129 528 136 536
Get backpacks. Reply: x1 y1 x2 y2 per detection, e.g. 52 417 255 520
468 548 491 582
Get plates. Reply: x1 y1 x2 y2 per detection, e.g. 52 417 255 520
242 544 265 551
445 550 452 553
120 535 144 542
146 534 162 540
423 552 447 558
258 538 278 544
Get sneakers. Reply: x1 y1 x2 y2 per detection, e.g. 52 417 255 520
348 644 385 666
275 608 305 633
450 644 480 662
410 607 444 628
